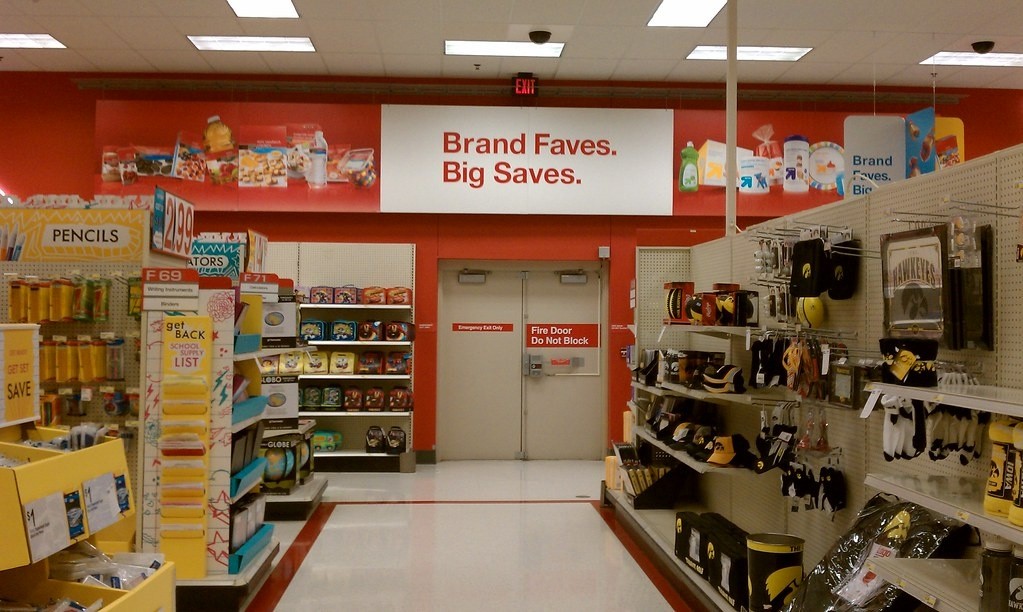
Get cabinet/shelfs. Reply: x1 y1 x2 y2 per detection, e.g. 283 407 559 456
2 269 416 612
628 312 771 484
853 368 1019 609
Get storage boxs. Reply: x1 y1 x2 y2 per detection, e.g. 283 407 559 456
600 410 755 612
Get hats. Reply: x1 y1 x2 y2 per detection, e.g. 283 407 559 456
656 365 752 465
